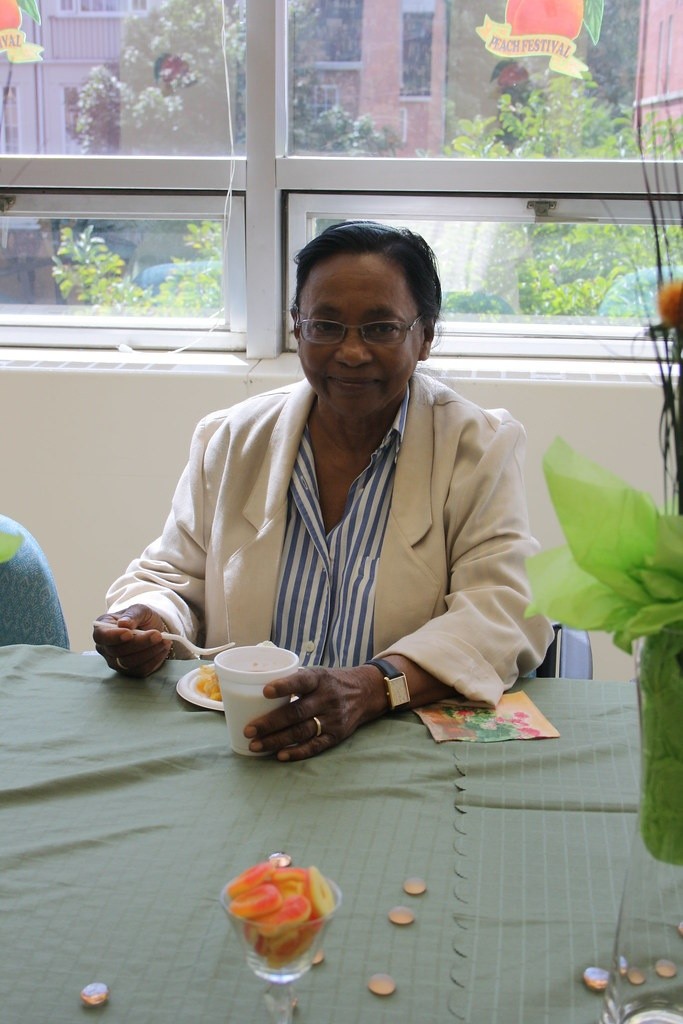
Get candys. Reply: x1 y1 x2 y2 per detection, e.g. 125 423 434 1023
224 861 337 967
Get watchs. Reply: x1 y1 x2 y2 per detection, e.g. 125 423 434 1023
361 658 412 715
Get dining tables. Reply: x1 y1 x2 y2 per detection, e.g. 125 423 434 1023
1 644 683 1024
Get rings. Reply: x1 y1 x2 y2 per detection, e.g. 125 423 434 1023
116 657 126 669
313 716 322 737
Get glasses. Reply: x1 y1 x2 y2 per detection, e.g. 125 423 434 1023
293 304 424 345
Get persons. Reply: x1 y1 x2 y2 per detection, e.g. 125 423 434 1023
91 219 556 764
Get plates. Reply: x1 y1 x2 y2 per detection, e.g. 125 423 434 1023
176 663 299 711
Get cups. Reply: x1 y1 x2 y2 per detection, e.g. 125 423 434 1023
221 872 343 984
214 646 300 757
